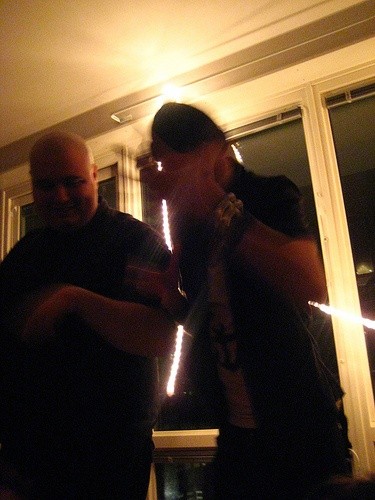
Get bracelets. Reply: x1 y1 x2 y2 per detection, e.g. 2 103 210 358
209 191 254 249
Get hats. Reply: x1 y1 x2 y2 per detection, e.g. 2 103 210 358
150 101 225 157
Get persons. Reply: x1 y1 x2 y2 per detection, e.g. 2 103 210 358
136 104 353 500
0 131 184 500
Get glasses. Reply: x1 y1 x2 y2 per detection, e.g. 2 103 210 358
32 173 94 190
